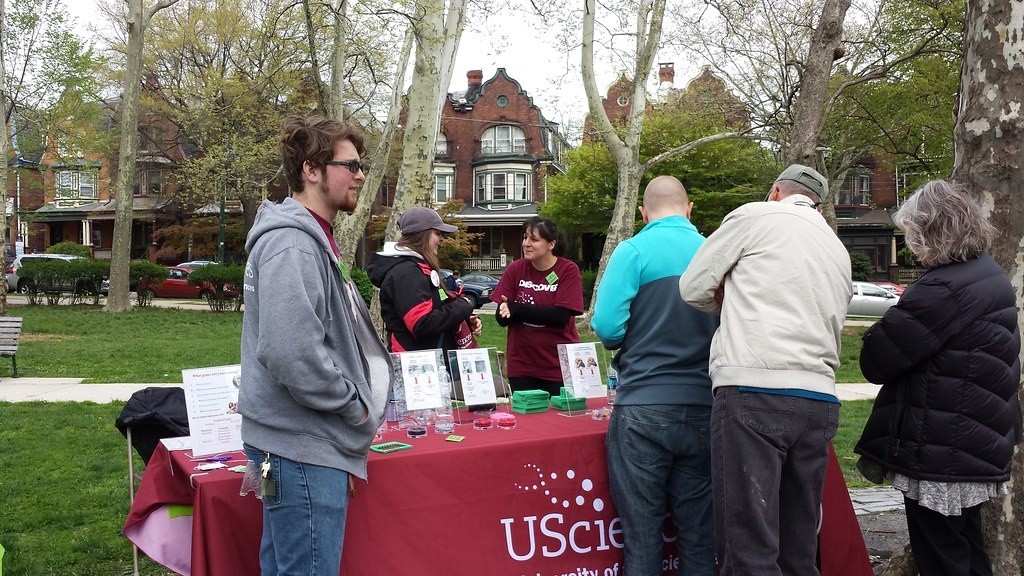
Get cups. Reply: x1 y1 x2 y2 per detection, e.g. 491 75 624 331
473 410 493 430
372 429 382 445
407 417 427 438
434 415 454 434
497 414 516 430
419 410 437 427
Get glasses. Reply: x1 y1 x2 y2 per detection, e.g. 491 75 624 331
325 159 366 171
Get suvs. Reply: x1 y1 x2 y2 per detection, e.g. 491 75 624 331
441 268 493 309
170 260 220 278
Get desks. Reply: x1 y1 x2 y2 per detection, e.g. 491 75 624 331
120 396 875 576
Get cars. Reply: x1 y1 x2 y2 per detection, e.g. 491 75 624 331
7 253 110 297
137 266 240 301
868 282 907 297
460 274 500 293
844 281 904 319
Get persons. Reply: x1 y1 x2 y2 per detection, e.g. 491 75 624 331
591 176 721 576
238 113 395 576
678 163 853 576
852 179 1020 576
445 270 460 292
366 206 482 353
488 217 585 399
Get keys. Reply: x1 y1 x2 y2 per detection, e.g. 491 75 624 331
260 462 270 478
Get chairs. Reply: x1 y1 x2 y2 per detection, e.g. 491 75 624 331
115 387 190 576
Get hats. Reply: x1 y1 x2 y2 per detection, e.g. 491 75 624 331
397 206 458 235
764 164 831 207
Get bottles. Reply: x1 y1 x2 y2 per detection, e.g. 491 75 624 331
435 366 453 415
607 363 618 405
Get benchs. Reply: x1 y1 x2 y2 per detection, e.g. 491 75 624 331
0 317 23 377
